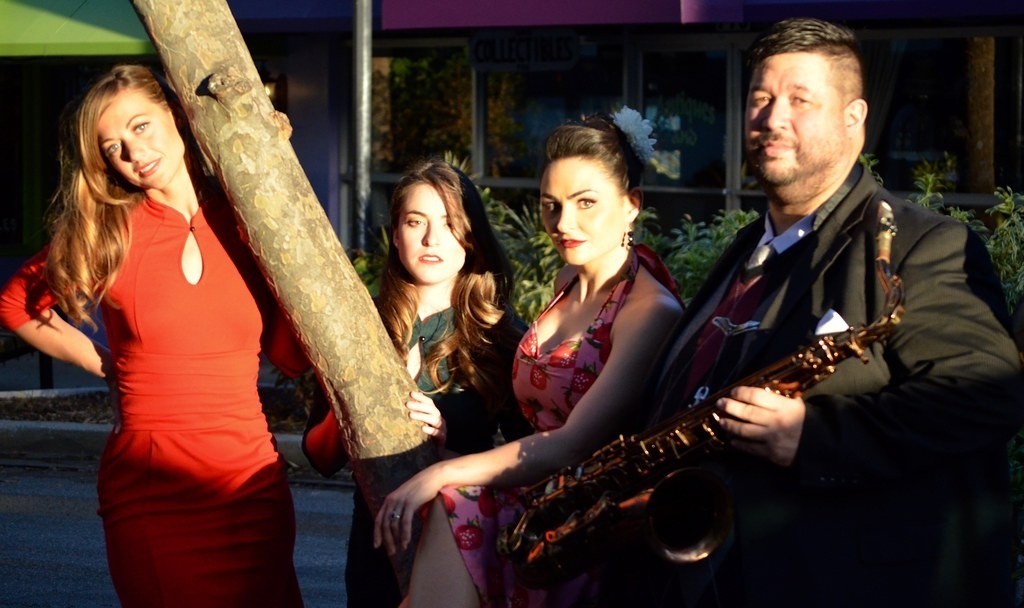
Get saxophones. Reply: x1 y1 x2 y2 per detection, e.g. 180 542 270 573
489 199 912 571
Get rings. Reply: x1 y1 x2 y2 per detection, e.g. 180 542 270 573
389 511 400 521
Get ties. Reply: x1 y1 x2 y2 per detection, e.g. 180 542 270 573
744 241 774 269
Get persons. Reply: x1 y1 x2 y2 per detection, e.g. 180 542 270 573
302 161 530 608
583 16 1024 608
372 104 684 608
0 64 313 608
